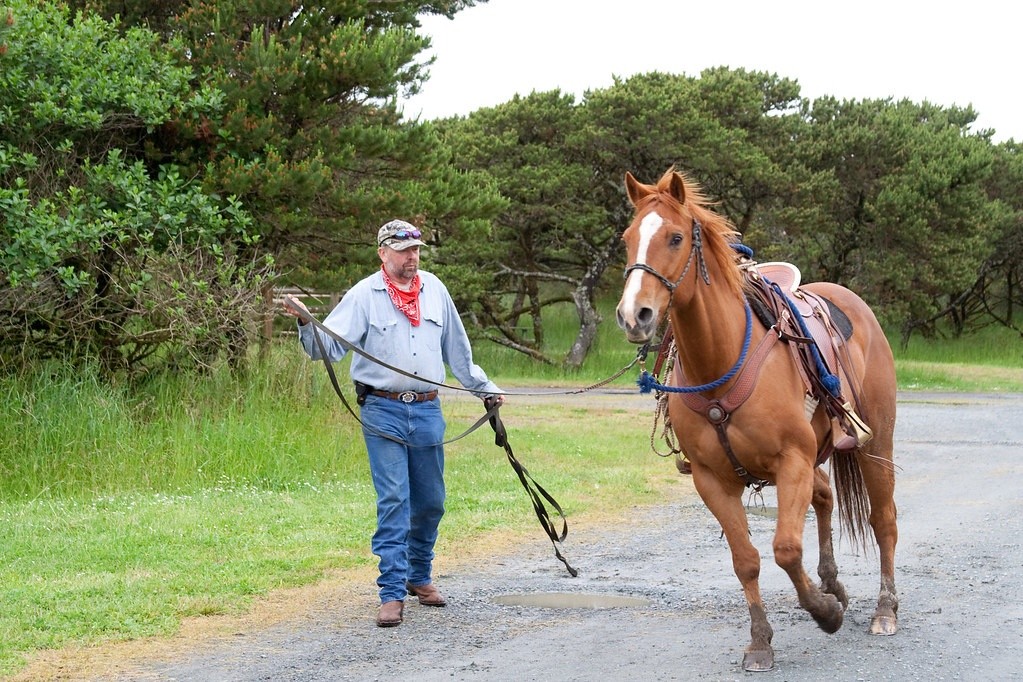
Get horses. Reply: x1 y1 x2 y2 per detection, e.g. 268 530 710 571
615 164 899 671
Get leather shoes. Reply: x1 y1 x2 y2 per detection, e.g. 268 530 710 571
406 581 446 607
376 600 404 626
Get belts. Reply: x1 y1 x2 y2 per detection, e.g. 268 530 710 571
369 388 438 402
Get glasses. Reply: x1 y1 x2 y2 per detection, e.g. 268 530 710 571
379 230 421 247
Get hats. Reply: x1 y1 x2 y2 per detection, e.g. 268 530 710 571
378 220 429 251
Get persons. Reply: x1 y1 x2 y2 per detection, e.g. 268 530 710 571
283 219 507 627
